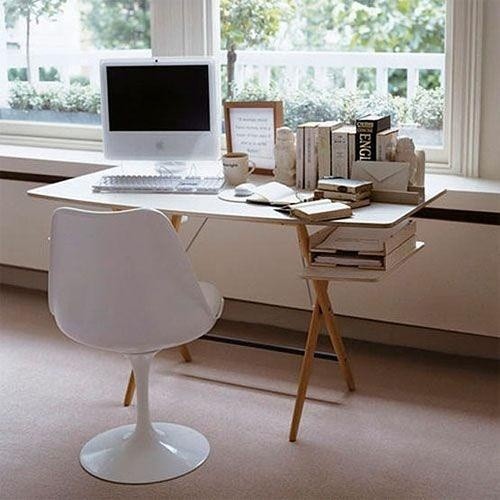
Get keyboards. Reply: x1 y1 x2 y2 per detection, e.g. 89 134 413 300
92 174 226 194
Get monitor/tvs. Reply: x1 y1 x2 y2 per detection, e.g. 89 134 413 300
100 56 222 172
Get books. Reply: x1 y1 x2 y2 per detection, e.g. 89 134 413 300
245 114 399 223
313 254 382 267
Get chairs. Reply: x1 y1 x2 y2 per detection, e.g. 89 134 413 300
46 206 226 484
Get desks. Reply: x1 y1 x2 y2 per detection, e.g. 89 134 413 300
27 163 449 446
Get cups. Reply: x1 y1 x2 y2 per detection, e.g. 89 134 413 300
222 153 256 187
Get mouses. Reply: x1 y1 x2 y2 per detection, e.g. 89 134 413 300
235 183 256 195
